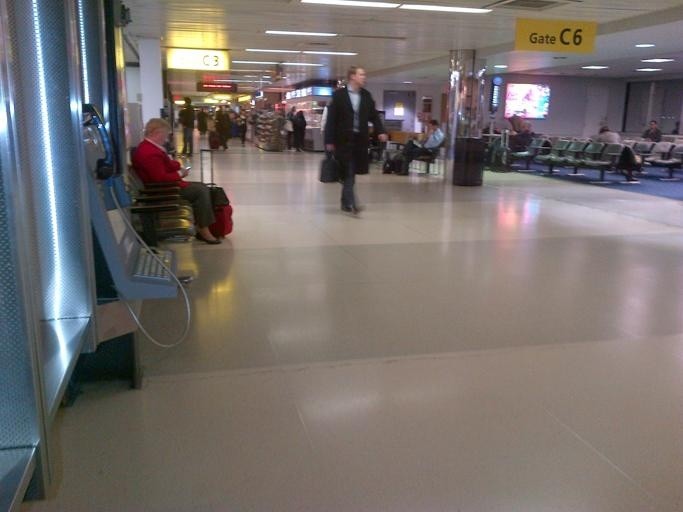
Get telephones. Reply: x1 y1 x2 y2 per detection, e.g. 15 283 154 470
79 104 120 181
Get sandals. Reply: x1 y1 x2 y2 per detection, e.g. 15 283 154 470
196 230 221 244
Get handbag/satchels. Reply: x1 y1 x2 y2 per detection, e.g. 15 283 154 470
199 149 230 205
320 151 340 182
383 159 392 174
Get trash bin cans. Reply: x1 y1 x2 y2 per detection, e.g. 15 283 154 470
453 137 484 186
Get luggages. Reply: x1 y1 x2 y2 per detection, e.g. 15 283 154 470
209 206 233 236
209 131 219 149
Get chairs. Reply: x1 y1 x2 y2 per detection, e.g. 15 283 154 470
510 136 683 186
122 164 199 301
390 138 446 173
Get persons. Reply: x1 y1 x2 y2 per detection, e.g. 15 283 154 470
520 121 533 136
324 65 388 217
285 107 294 151
394 120 444 176
292 111 306 152
593 125 621 145
641 121 661 143
131 117 220 245
175 96 248 157
